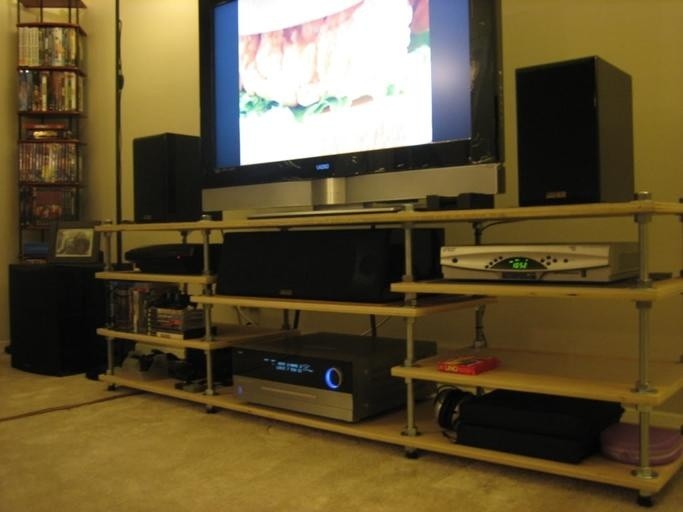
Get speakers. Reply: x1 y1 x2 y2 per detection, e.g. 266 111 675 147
133 132 204 224
516 55 635 207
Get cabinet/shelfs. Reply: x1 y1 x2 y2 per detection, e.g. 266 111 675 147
16 0 87 264
92 191 683 507
5 263 110 376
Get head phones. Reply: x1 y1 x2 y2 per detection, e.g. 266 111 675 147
433 384 476 431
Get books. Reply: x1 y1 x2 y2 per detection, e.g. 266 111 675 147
112 283 219 339
17 24 84 265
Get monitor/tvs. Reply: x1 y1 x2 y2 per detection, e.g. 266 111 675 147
198 0 506 219
